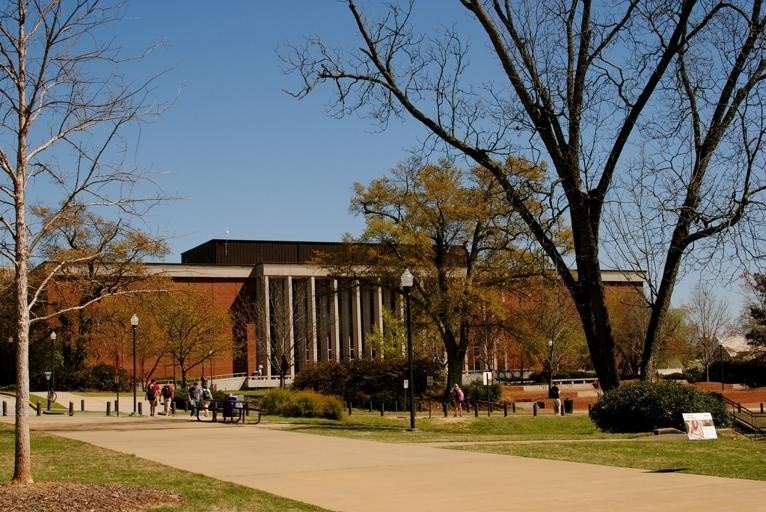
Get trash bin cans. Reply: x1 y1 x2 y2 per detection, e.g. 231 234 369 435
564 400 573 413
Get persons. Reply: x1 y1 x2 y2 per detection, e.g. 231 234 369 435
223 393 245 422
199 382 213 417
145 377 161 415
597 381 602 393
159 380 174 417
686 420 704 439
187 382 201 422
551 382 563 416
450 383 465 418
592 380 597 393
256 360 264 379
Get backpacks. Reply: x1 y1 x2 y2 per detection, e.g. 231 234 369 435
202 389 210 400
163 386 171 398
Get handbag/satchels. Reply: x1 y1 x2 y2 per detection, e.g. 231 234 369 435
147 389 154 400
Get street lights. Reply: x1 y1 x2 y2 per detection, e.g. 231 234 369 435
718 337 726 391
546 340 554 391
129 313 139 413
7 337 14 388
50 330 56 393
209 349 213 387
44 371 53 407
399 267 418 430
